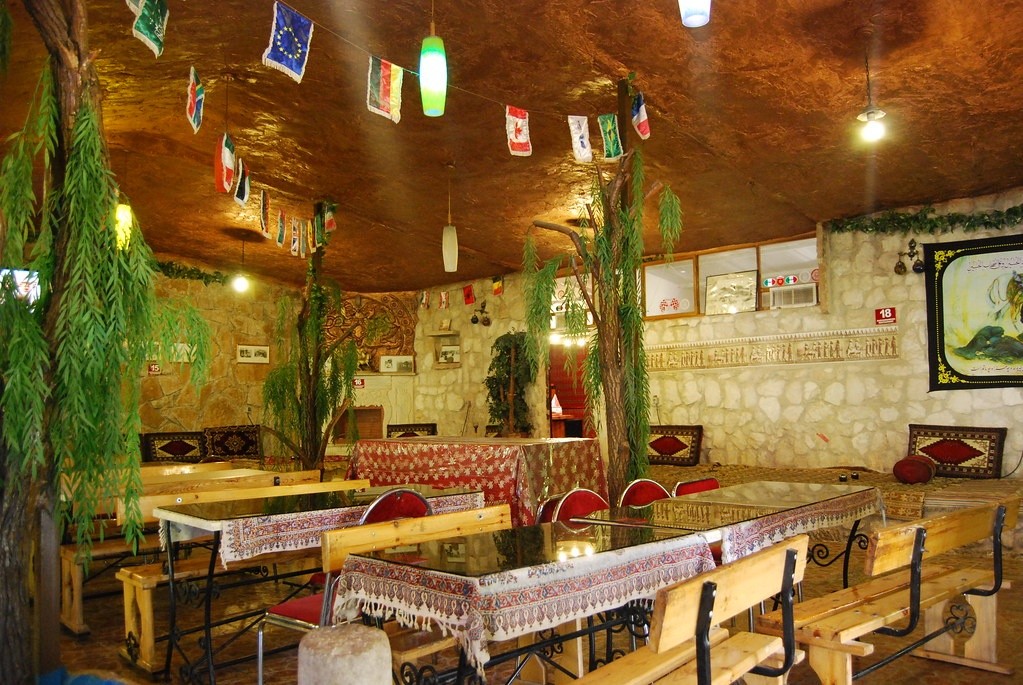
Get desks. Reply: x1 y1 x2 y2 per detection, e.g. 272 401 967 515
332 521 716 685
345 435 609 528
568 480 886 659
153 484 485 685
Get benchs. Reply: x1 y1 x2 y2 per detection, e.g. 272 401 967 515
26 460 1022 685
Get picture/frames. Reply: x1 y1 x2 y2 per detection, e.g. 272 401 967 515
378 355 414 373
438 345 461 363
236 343 270 364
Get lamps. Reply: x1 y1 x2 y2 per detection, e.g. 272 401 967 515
678 0 711 27
856 27 887 143
893 236 923 277
419 0 447 118
115 153 132 251
231 232 250 291
442 164 459 272
469 301 491 326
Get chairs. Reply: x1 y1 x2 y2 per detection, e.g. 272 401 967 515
671 477 765 626
552 488 610 522
617 478 672 652
257 487 434 685
